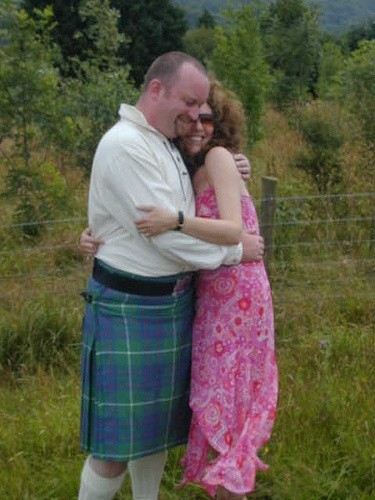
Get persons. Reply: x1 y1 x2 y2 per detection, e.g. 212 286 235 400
80 72 278 500
77 52 265 500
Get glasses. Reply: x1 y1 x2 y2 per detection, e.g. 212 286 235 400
193 114 214 125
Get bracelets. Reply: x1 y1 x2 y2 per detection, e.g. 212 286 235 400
175 211 184 233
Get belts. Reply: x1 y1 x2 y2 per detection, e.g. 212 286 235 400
92 260 195 297
